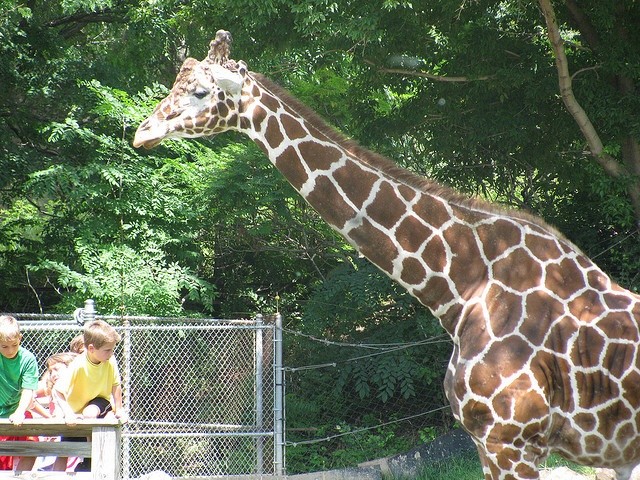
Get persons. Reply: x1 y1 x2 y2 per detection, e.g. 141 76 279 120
51 318 126 471
0 315 40 472
29 370 56 420
70 334 84 353
31 352 79 419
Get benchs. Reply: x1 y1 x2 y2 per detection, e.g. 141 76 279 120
1 420 125 480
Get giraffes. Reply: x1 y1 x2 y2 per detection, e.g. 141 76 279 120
133 29 640 480
